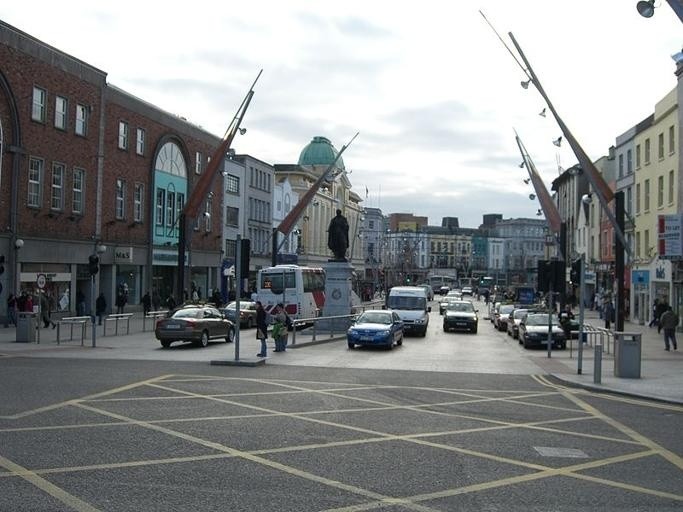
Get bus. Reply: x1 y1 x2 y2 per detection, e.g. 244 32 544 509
255 264 326 327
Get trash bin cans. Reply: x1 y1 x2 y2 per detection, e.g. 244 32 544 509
615 332 642 380
17 310 36 344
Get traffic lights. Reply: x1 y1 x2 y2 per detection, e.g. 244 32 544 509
87 255 98 274
570 259 581 284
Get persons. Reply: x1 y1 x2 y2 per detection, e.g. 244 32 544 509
254 299 269 358
1 283 254 329
271 316 286 353
275 302 289 349
326 208 350 260
472 283 680 352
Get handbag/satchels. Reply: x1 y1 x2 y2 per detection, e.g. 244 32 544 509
286 317 294 326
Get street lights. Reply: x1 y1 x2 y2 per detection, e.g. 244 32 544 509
635 0 681 28
508 28 629 340
512 130 567 312
177 68 264 303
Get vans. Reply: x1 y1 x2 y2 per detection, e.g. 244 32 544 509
386 286 432 337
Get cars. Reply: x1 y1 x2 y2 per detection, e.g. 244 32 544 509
438 286 480 333
220 301 260 329
489 302 567 350
155 305 234 346
347 310 404 350
